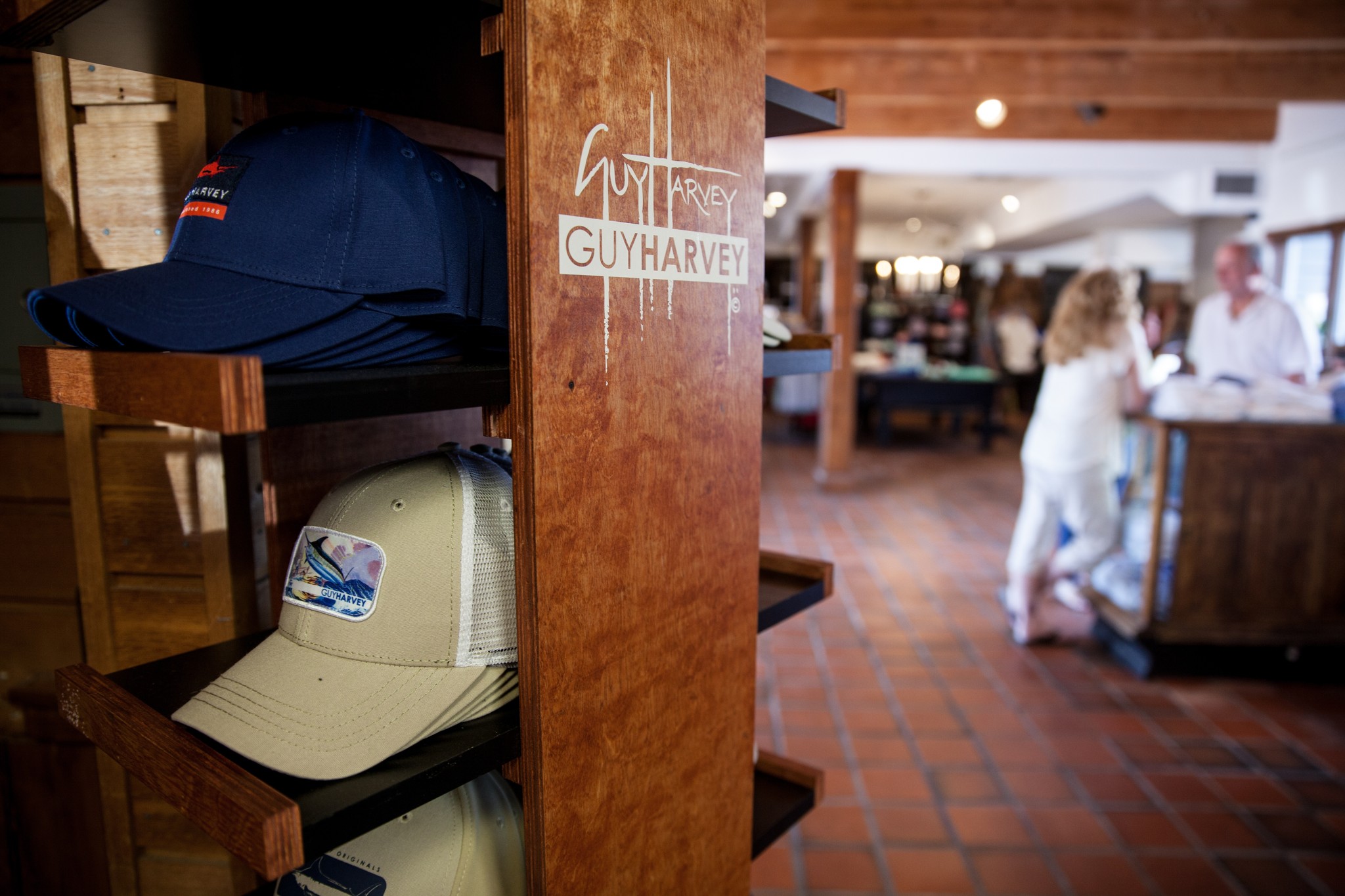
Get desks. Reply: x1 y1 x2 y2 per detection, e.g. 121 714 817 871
855 357 1001 455
1080 407 1345 664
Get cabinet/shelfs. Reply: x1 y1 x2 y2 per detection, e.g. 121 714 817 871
0 0 835 896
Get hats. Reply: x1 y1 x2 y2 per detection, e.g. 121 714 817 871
172 442 522 894
22 103 506 384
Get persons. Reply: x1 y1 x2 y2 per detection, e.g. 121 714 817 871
1318 344 1344 423
1001 261 1181 646
996 300 1170 623
1184 238 1325 390
993 293 1043 440
